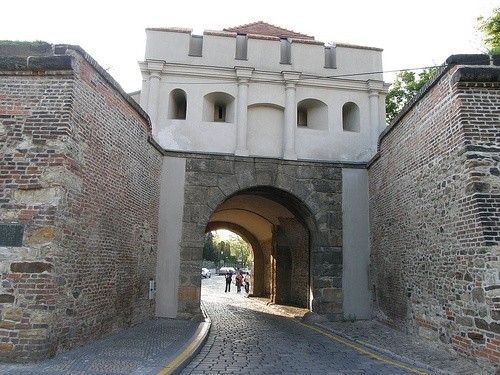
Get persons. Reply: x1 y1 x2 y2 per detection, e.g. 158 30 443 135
224 270 232 292
235 271 243 293
243 272 250 293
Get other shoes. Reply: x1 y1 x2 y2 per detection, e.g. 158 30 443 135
225 290 227 292
228 290 230 292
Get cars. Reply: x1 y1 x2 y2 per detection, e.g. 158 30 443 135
201 267 211 278
239 267 251 275
219 266 236 275
